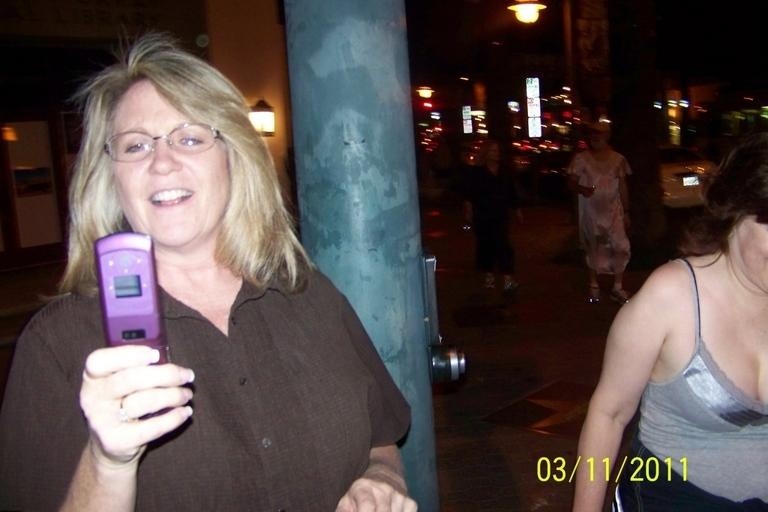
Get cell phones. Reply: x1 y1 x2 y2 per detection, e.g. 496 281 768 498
94 231 177 421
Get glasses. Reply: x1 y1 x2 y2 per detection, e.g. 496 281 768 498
103 121 222 163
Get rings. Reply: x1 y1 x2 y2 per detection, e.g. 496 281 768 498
116 393 137 425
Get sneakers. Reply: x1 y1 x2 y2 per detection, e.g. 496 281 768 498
482 274 519 292
586 285 630 305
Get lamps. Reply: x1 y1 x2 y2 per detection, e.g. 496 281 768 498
248 98 276 136
506 0 548 24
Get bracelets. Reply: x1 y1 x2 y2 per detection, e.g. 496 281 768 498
623 208 630 214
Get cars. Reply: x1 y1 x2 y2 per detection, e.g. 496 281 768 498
659 145 713 214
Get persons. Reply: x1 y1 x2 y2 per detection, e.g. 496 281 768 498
0 33 420 512
565 124 634 305
569 127 767 512
464 140 526 290
